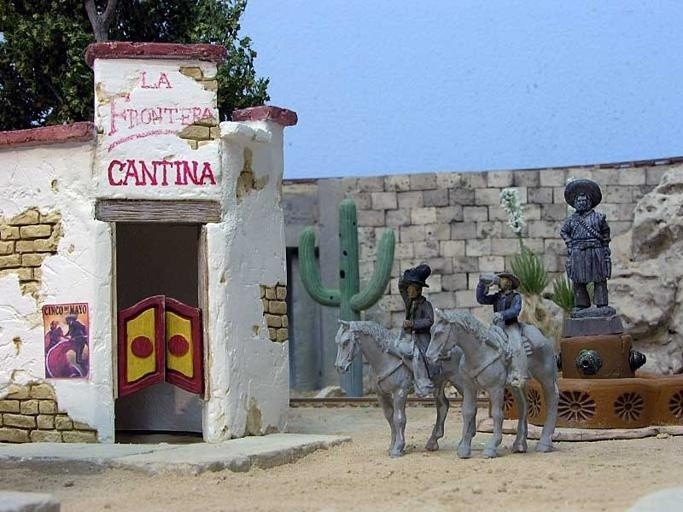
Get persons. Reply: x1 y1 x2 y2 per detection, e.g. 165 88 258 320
61 312 87 369
395 263 437 398
475 270 529 390
557 178 613 310
45 320 61 352
